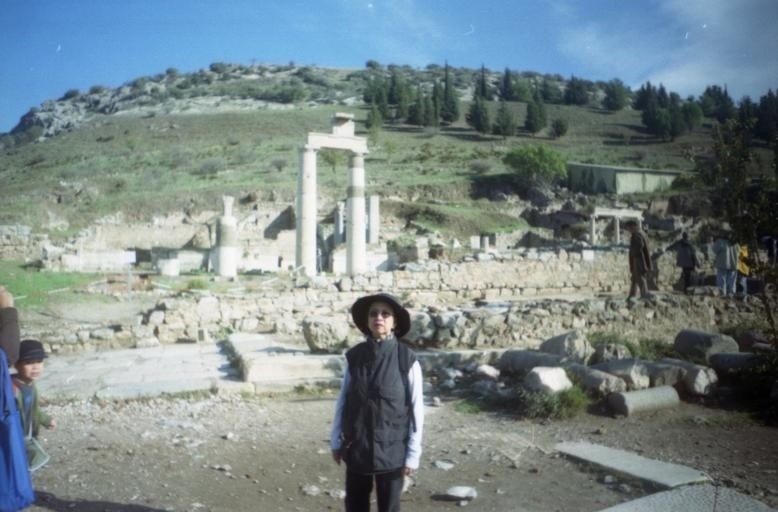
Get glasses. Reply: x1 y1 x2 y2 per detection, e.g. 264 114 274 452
368 309 393 319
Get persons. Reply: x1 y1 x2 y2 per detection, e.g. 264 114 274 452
0 286 21 366
667 233 699 294
329 293 426 511
733 239 750 297
623 220 650 300
714 230 741 299
10 339 57 472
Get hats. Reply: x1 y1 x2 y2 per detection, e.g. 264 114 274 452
351 293 412 338
18 339 48 362
718 222 734 232
